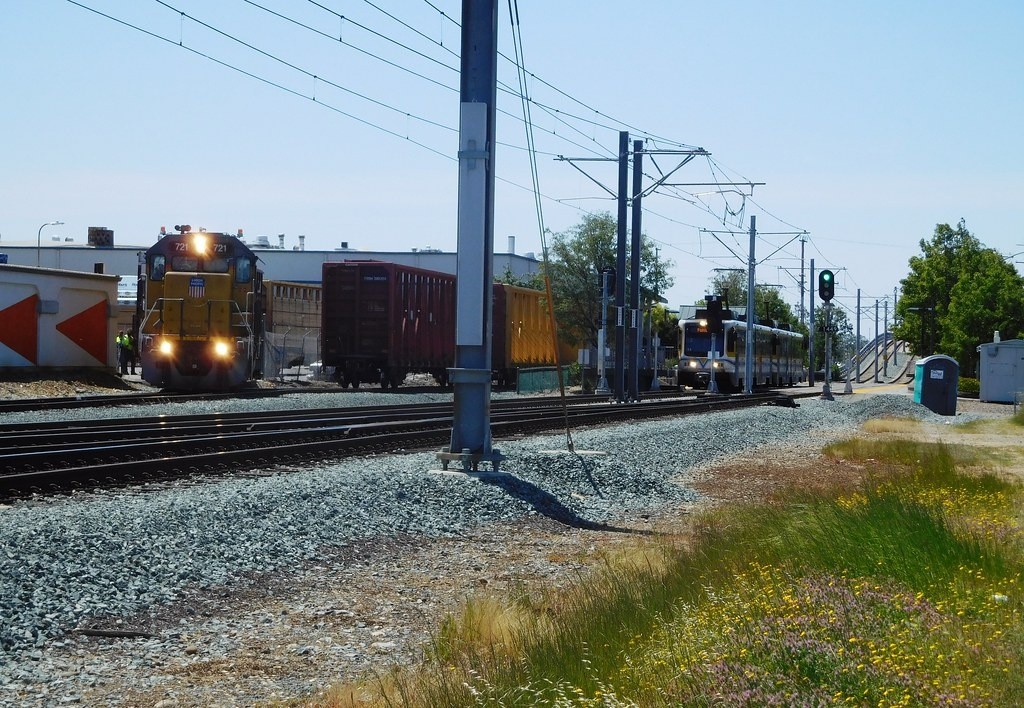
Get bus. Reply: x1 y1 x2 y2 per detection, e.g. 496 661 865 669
677 268 804 392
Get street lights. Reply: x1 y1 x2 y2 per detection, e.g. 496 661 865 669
38 220 65 267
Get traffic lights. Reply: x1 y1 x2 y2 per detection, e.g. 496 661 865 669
819 269 835 300
706 301 722 333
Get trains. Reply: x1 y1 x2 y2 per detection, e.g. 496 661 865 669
132 225 323 391
322 258 616 389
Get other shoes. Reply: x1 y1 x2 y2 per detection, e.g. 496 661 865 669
131 373 137 375
123 372 129 375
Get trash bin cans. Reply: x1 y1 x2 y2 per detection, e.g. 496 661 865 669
913 354 959 416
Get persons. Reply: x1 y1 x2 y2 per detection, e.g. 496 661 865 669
116 328 138 375
